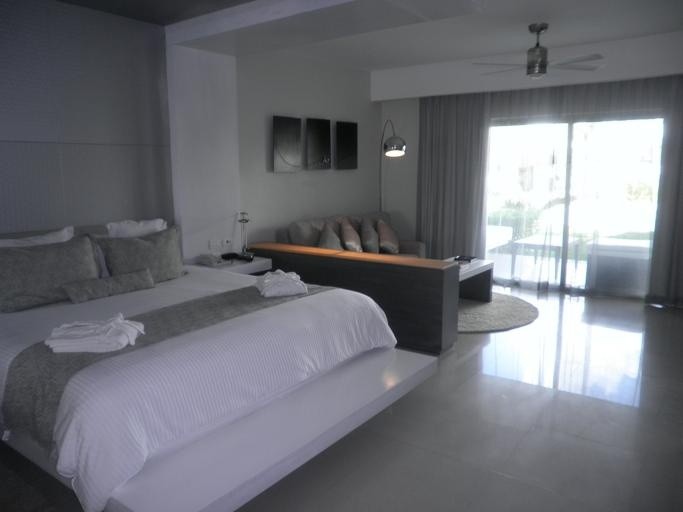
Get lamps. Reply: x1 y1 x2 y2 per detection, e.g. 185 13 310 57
379 119 407 210
525 22 549 76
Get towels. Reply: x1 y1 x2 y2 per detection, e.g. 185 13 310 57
255 268 308 298
43 311 146 353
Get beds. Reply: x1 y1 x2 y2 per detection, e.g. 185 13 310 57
1 268 438 512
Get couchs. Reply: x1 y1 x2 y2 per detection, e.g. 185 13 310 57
274 210 427 258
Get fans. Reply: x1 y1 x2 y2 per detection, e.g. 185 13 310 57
473 54 604 77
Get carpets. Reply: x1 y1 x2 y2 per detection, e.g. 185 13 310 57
458 291 539 333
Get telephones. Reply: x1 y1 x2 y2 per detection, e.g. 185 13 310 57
197 254 231 267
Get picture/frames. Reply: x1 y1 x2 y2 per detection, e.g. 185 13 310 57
272 114 302 173
336 120 358 170
305 117 331 170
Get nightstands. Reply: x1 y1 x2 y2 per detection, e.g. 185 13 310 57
206 254 272 276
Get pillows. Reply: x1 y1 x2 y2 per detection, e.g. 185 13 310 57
0 224 74 247
376 219 400 254
97 224 190 283
360 216 380 254
0 233 104 313
106 218 168 239
62 268 154 303
319 223 344 251
340 216 363 252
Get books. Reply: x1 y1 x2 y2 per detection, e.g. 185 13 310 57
452 255 479 271
